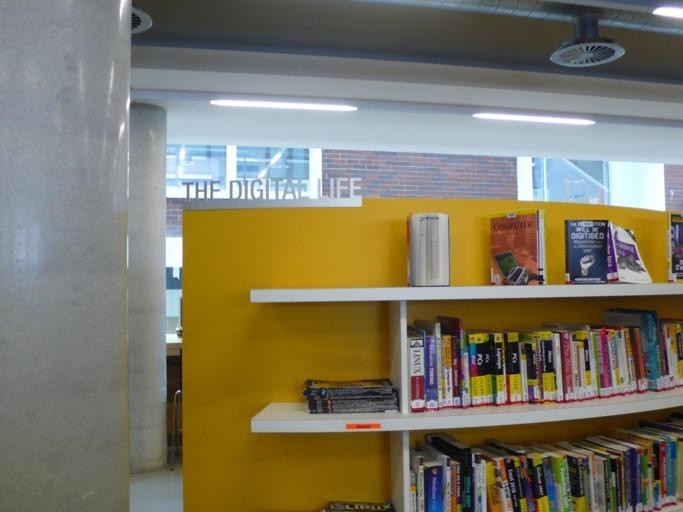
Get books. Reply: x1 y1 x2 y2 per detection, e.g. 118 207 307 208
409 415 682 511
321 502 396 511
606 221 652 284
564 218 608 285
301 378 400 414
666 211 682 284
490 208 548 287
407 307 682 413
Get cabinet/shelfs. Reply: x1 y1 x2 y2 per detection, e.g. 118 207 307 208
243 275 683 510
